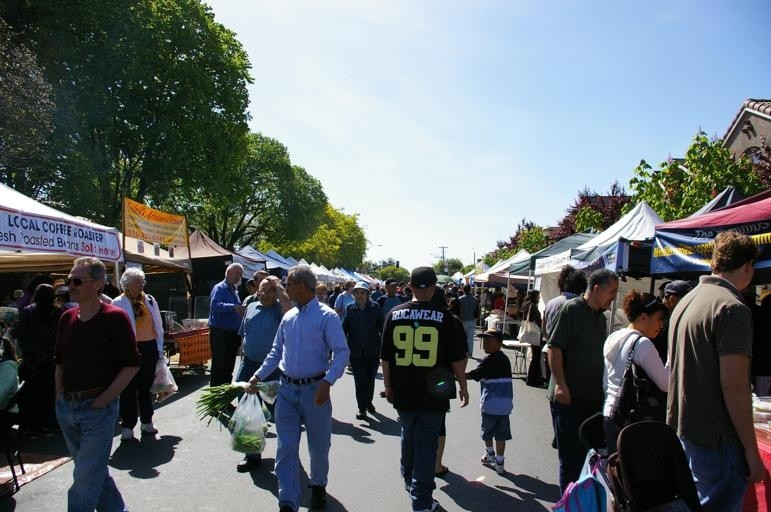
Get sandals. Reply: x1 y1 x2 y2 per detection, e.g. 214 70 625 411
434 464 449 477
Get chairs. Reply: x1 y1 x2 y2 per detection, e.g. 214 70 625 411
0 379 27 490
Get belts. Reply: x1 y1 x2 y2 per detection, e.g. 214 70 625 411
61 388 104 400
280 370 327 384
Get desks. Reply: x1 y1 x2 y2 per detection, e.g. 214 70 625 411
502 339 531 381
744 423 771 511
164 327 212 372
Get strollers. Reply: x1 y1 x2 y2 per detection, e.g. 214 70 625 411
578 408 705 512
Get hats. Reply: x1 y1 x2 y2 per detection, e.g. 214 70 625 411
351 281 372 296
385 278 398 288
410 266 438 289
662 279 692 297
476 328 504 342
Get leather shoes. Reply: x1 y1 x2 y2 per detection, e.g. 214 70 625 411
236 452 262 472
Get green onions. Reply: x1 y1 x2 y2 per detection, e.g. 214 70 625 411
195 382 277 431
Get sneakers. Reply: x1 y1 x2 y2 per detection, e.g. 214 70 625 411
140 422 159 433
120 426 135 440
23 428 57 440
355 402 377 419
479 448 506 474
306 484 328 512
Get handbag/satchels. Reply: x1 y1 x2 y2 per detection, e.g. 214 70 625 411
516 302 542 347
548 453 608 512
608 334 668 434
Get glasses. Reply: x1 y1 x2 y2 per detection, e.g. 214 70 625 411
64 276 97 286
643 295 663 308
